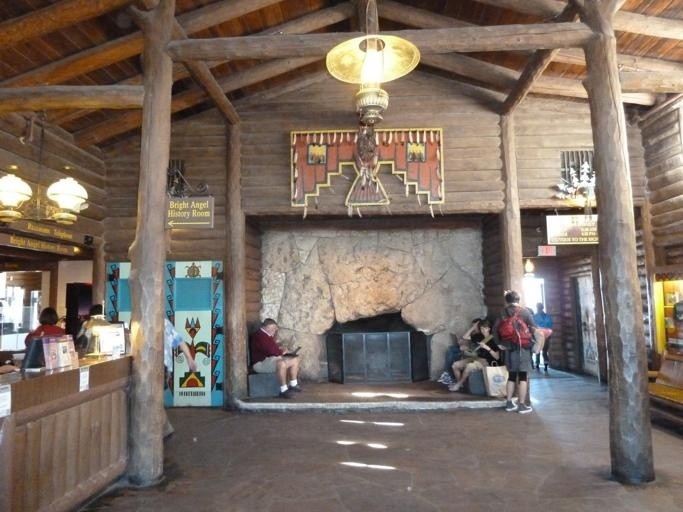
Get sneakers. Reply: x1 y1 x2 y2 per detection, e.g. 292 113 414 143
518 402 533 413
506 399 517 412
280 390 294 398
437 371 464 392
289 385 307 392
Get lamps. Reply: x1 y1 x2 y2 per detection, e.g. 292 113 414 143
0 112 89 225
325 0 421 125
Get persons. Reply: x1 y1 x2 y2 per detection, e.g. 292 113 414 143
436 317 481 385
25 308 66 347
249 318 302 399
447 321 500 391
496 290 536 414
532 302 553 371
164 318 196 406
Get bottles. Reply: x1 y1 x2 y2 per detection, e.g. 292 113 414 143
113 334 121 357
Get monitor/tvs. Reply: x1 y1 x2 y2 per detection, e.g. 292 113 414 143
20 337 42 370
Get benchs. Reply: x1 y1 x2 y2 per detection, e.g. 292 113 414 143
645 350 683 425
446 345 488 396
249 372 280 397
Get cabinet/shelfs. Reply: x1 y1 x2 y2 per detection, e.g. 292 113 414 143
1 286 32 352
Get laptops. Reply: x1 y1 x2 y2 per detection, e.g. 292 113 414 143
285 347 301 355
450 333 460 348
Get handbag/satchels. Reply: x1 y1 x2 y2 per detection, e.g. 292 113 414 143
463 350 477 362
498 316 553 355
482 364 509 397
76 335 87 351
